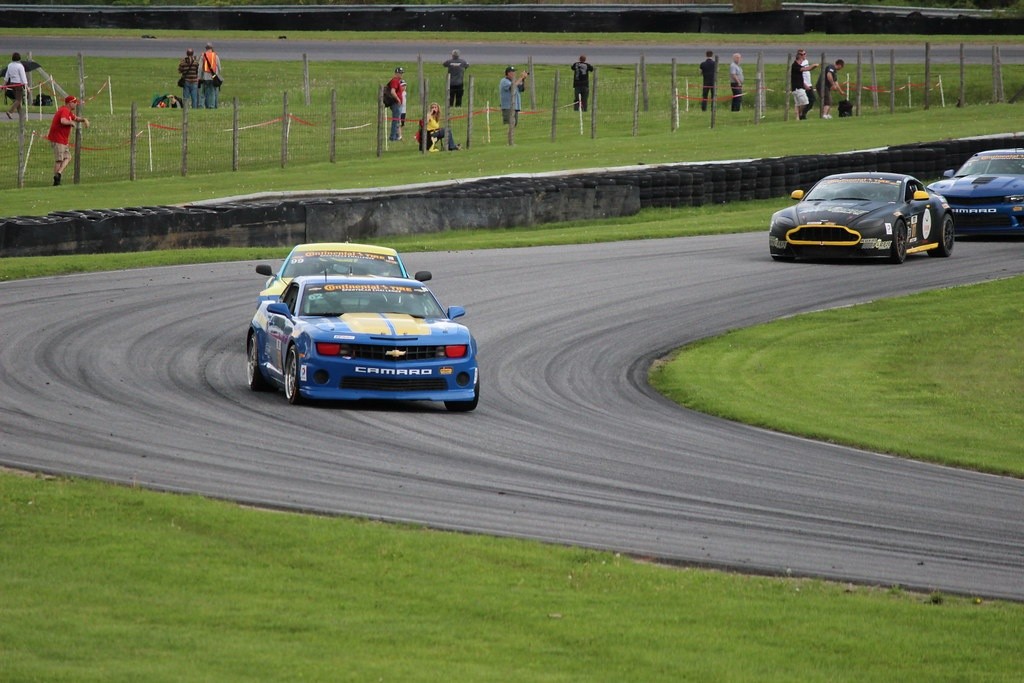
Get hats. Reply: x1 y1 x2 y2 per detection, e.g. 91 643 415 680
506 66 517 72
400 80 406 85
395 67 405 73
65 95 80 103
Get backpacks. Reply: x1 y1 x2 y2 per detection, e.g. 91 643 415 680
838 99 852 117
384 81 395 106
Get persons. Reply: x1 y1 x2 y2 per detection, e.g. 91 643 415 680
730 53 744 112
798 49 816 120
387 67 407 140
791 54 818 120
427 103 460 150
816 59 845 120
500 66 527 146
168 43 221 109
2 53 30 119
49 96 89 185
444 49 469 106
571 56 593 111
699 51 715 111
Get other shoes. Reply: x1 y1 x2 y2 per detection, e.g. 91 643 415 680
6 110 13 119
823 114 832 119
54 173 61 185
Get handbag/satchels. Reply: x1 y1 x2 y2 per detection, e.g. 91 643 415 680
178 79 185 87
4 87 16 104
212 73 223 86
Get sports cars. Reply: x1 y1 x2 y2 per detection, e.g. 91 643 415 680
925 148 1024 237
255 242 433 310
768 171 955 264
245 276 480 411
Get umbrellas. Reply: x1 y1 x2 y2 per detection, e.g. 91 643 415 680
0 61 41 77
